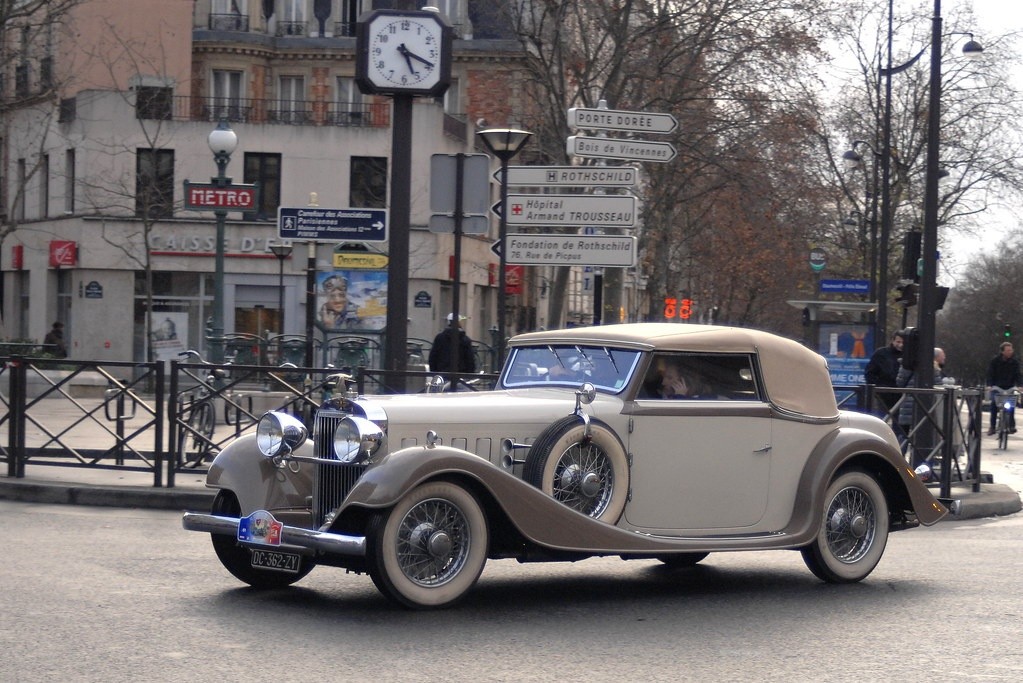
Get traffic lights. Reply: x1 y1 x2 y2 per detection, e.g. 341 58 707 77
489 234 634 267
1005 323 1011 337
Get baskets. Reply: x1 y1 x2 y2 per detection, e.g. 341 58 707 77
994 393 1018 408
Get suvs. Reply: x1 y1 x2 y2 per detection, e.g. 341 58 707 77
183 322 963 612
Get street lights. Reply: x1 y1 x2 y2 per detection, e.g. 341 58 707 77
868 30 983 348
475 126 534 369
842 140 879 302
207 111 238 424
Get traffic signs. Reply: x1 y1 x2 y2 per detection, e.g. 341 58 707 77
490 194 635 226
575 107 678 133
490 166 638 186
277 206 389 242
574 136 677 162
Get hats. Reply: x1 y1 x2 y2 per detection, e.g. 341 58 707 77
52 322 64 328
447 313 468 320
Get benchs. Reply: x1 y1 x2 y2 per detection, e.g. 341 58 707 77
717 389 755 400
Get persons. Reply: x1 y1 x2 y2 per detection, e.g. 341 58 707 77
987 342 1023 436
662 358 717 400
932 347 958 384
429 312 475 373
43 322 68 358
320 276 362 329
896 326 919 455
864 330 904 420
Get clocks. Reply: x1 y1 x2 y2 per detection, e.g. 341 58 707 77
367 15 443 90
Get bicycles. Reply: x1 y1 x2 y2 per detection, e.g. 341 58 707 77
986 385 1023 451
177 350 237 470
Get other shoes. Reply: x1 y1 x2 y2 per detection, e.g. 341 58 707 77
987 427 995 436
1009 428 1017 434
